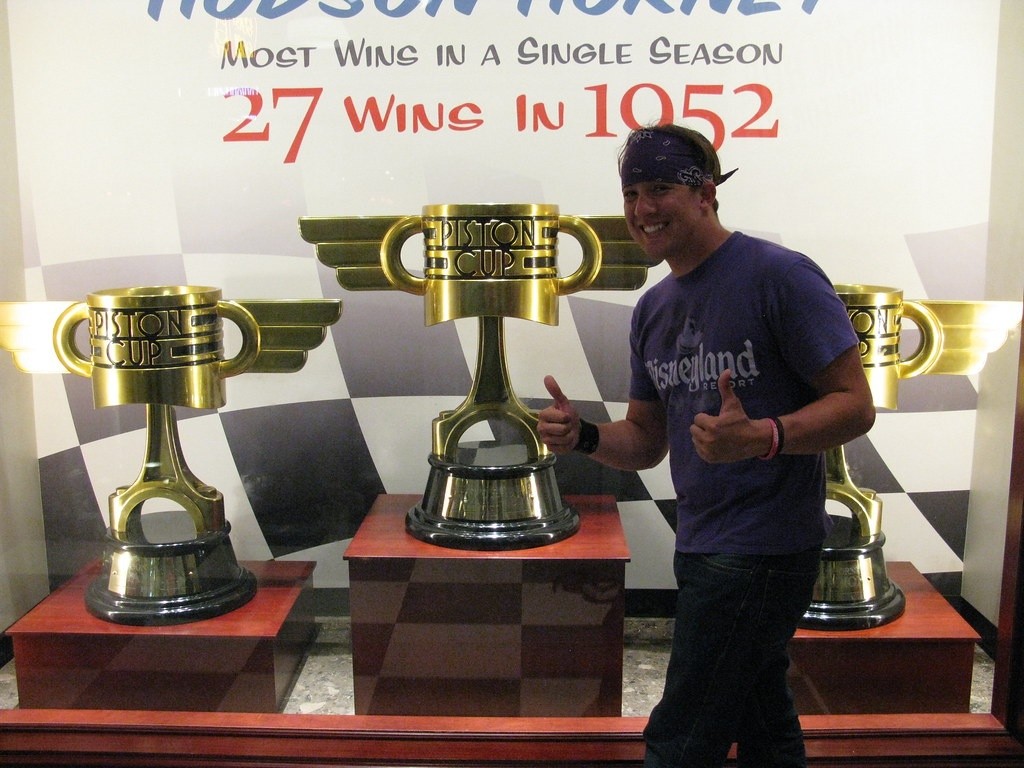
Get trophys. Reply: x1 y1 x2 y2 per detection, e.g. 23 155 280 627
1 285 342 626
797 287 1020 633
298 203 667 549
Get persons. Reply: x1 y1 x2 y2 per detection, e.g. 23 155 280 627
538 123 879 768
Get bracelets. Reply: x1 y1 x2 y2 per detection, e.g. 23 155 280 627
757 416 784 461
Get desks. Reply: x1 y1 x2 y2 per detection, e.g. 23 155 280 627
339 495 633 716
783 562 982 717
3 553 321 714
